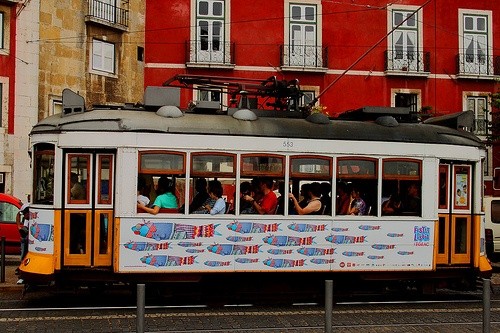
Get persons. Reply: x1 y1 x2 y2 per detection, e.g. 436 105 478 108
194 180 226 214
457 185 468 205
382 184 421 215
288 181 332 215
180 178 208 214
16 193 32 283
336 181 376 217
138 176 178 215
136 179 151 206
230 178 284 214
69 172 86 200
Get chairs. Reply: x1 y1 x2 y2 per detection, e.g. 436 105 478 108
136 202 372 215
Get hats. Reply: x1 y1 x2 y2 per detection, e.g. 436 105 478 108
20 204 29 211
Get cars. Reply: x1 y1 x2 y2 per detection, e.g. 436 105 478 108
0 193 25 256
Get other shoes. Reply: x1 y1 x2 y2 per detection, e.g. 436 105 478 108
17 279 24 284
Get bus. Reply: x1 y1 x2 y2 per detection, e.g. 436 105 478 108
16 0 494 309
